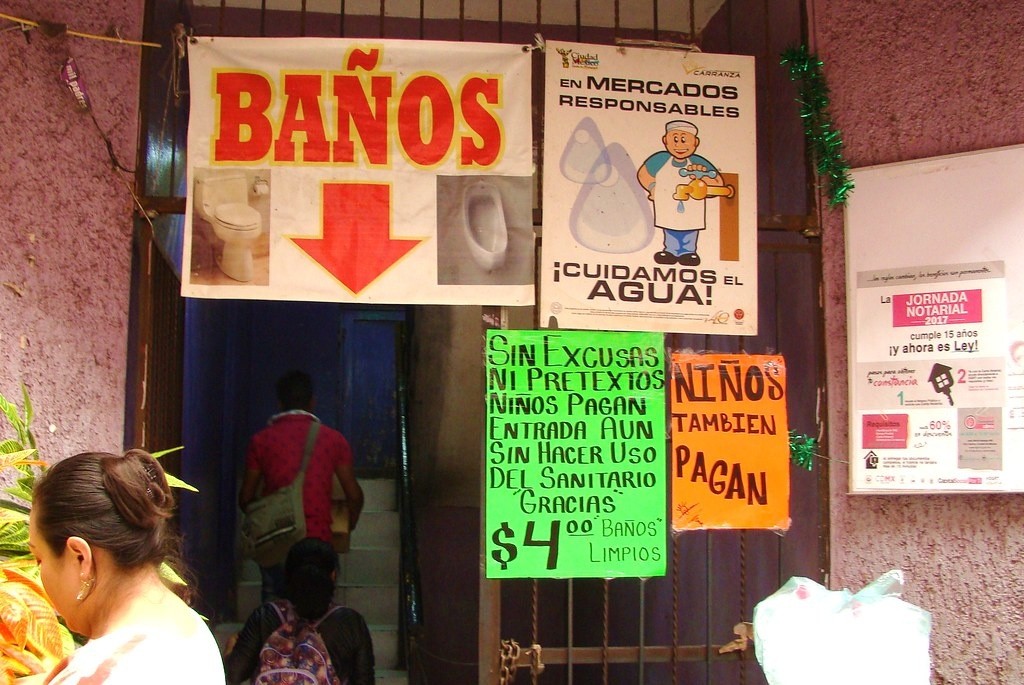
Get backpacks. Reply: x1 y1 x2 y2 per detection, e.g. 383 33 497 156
253 598 345 685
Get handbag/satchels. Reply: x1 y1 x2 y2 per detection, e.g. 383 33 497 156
236 485 306 565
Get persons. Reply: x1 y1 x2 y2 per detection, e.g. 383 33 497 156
15 448 229 685
223 536 376 685
236 368 363 614
